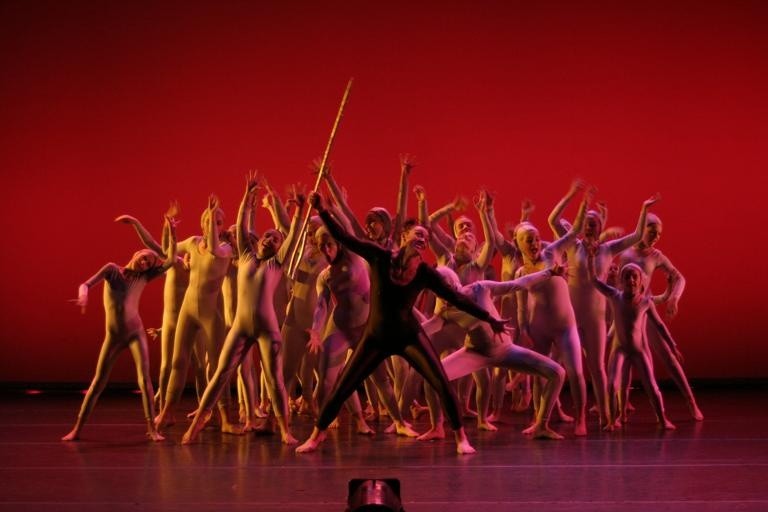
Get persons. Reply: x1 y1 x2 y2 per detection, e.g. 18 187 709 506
61 150 706 458
